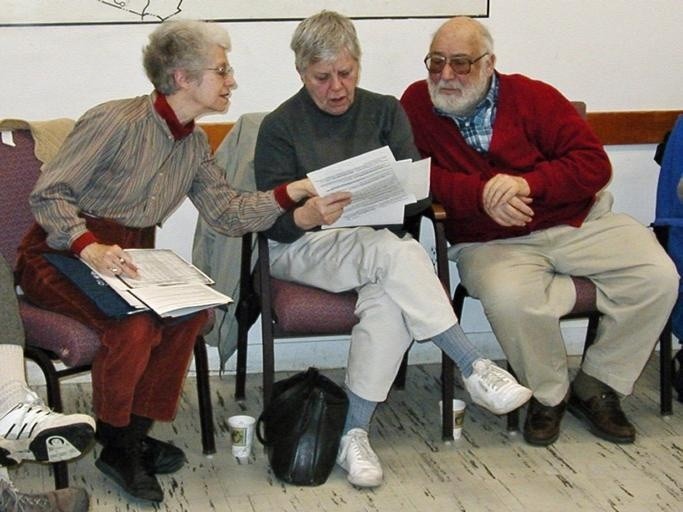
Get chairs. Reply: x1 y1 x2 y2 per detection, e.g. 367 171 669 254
425 100 608 439
0 117 219 491
235 112 460 450
648 107 682 420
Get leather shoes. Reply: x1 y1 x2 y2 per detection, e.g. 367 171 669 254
525 390 566 445
569 381 636 444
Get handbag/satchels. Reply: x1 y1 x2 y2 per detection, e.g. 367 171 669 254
255 368 350 487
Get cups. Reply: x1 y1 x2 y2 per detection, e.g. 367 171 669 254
227 415 256 460
438 398 465 439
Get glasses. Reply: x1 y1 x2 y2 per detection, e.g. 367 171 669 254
204 65 235 77
424 52 491 73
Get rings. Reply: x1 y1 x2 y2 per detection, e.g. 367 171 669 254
109 264 118 273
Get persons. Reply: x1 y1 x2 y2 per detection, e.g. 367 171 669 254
14 13 318 502
253 9 534 489
0 251 98 493
399 13 682 445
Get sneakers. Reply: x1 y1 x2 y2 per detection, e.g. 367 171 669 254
334 428 385 490
461 359 533 417
95 421 187 502
2 385 96 465
